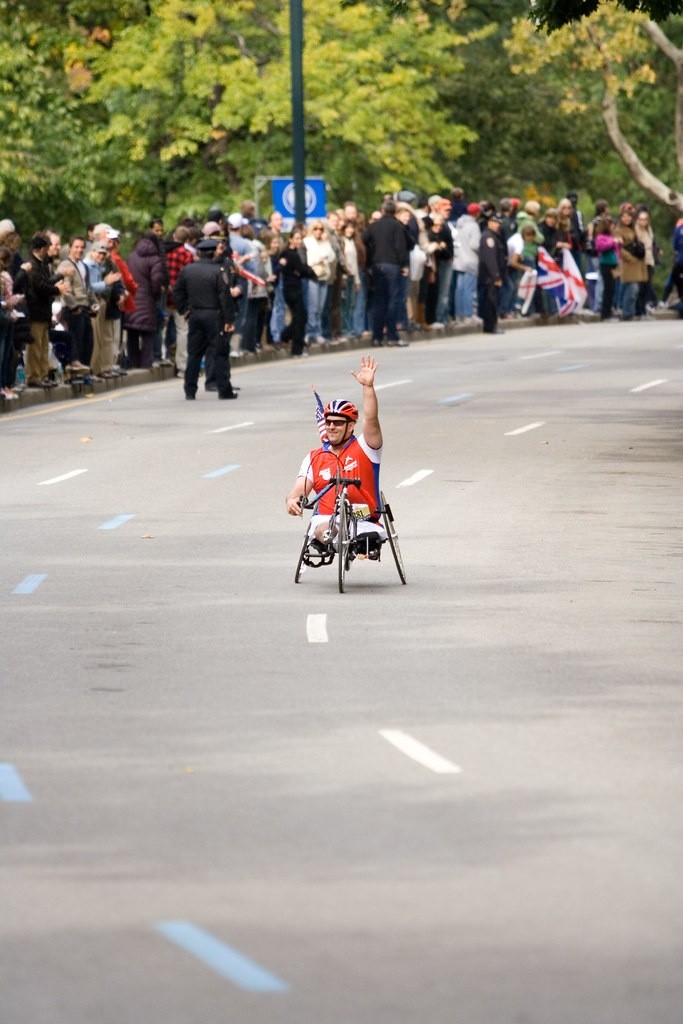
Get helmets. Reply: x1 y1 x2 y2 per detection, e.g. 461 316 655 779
324 399 359 422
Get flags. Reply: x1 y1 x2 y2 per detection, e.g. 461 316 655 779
313 391 328 444
512 242 589 318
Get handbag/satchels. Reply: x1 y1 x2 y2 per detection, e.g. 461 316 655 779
598 249 619 267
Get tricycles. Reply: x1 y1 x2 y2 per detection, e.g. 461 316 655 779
293 475 408 594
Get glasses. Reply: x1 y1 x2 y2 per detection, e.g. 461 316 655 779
326 419 353 426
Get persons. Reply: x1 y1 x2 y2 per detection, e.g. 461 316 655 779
284 353 384 560
1 190 683 400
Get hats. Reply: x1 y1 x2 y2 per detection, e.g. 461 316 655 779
195 240 217 251
511 197 522 208
467 203 481 214
93 243 109 253
107 229 122 240
489 211 503 224
399 190 417 203
203 222 221 235
228 213 249 228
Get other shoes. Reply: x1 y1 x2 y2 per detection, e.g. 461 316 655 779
218 392 238 399
29 378 58 388
152 358 174 368
233 299 682 359
232 387 240 390
186 393 195 400
71 375 95 384
97 367 128 378
206 385 218 391
64 364 90 374
0 386 20 400
